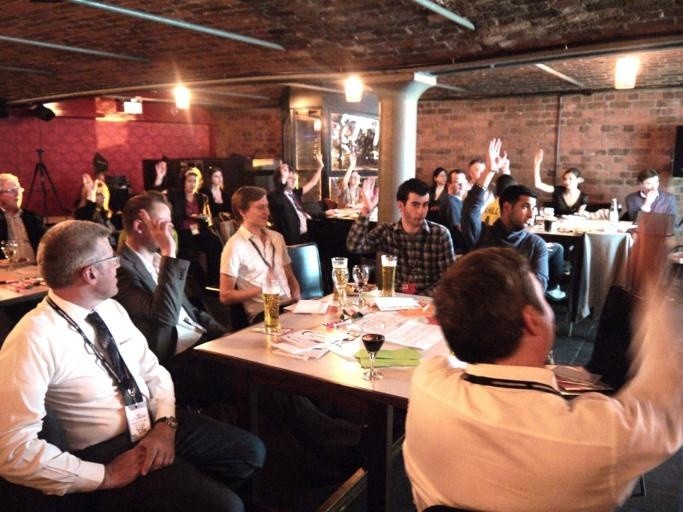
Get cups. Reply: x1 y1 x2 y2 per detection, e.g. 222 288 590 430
379 254 398 298
260 278 282 334
543 213 552 232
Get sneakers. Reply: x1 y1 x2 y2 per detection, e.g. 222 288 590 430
546 284 568 302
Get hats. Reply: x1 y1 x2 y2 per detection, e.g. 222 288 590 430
84 313 142 404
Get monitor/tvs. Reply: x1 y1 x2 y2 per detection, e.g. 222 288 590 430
671 125 683 178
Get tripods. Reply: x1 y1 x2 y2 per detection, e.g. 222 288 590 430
23 149 65 223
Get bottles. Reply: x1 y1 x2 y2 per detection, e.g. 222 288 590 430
608 198 619 233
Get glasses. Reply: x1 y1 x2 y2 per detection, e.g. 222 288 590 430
0 186 25 194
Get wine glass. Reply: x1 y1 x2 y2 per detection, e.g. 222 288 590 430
359 322 387 381
0 239 17 272
330 254 371 312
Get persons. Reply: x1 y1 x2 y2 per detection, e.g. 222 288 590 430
0 137 682 512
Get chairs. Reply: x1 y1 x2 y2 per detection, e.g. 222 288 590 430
584 286 651 497
286 242 323 299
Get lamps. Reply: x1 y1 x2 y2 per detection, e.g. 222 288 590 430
614 70 635 90
175 96 190 110
344 87 363 104
